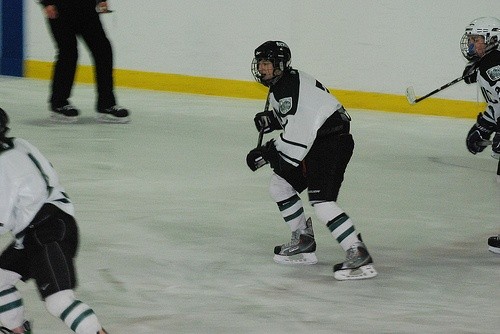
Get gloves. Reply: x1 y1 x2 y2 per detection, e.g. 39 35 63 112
463 66 477 84
254 110 285 134
246 141 278 171
466 112 498 155
492 116 500 153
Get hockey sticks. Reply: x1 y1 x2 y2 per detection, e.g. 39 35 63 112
255 81 274 149
405 69 478 106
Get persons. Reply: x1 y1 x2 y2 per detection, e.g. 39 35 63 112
0 107 108 334
246 40 379 273
459 16 500 248
35 0 129 118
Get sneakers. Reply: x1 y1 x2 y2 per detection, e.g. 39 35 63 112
272 218 318 264
488 234 500 254
49 102 78 119
96 105 129 123
333 233 378 280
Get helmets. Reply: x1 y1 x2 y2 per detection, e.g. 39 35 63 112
0 108 10 134
255 40 292 72
465 16 500 52
